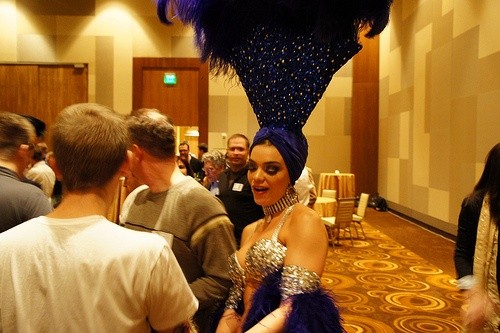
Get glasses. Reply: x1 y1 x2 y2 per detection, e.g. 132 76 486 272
202 165 216 172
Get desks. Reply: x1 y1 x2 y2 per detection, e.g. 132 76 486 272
318 172 355 199
313 196 338 218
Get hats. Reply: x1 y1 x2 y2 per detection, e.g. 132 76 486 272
249 126 309 186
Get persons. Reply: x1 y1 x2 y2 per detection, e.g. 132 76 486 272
454 142 500 330
175 134 227 186
211 134 264 249
210 123 345 333
0 103 200 333
0 111 62 233
118 108 239 333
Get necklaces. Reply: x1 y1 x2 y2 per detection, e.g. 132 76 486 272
263 193 299 224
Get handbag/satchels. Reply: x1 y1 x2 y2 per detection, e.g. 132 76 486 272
374 196 388 212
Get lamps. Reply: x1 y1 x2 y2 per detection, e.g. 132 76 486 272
184 126 199 137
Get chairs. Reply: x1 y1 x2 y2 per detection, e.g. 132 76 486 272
320 200 354 251
342 193 370 240
322 190 337 200
